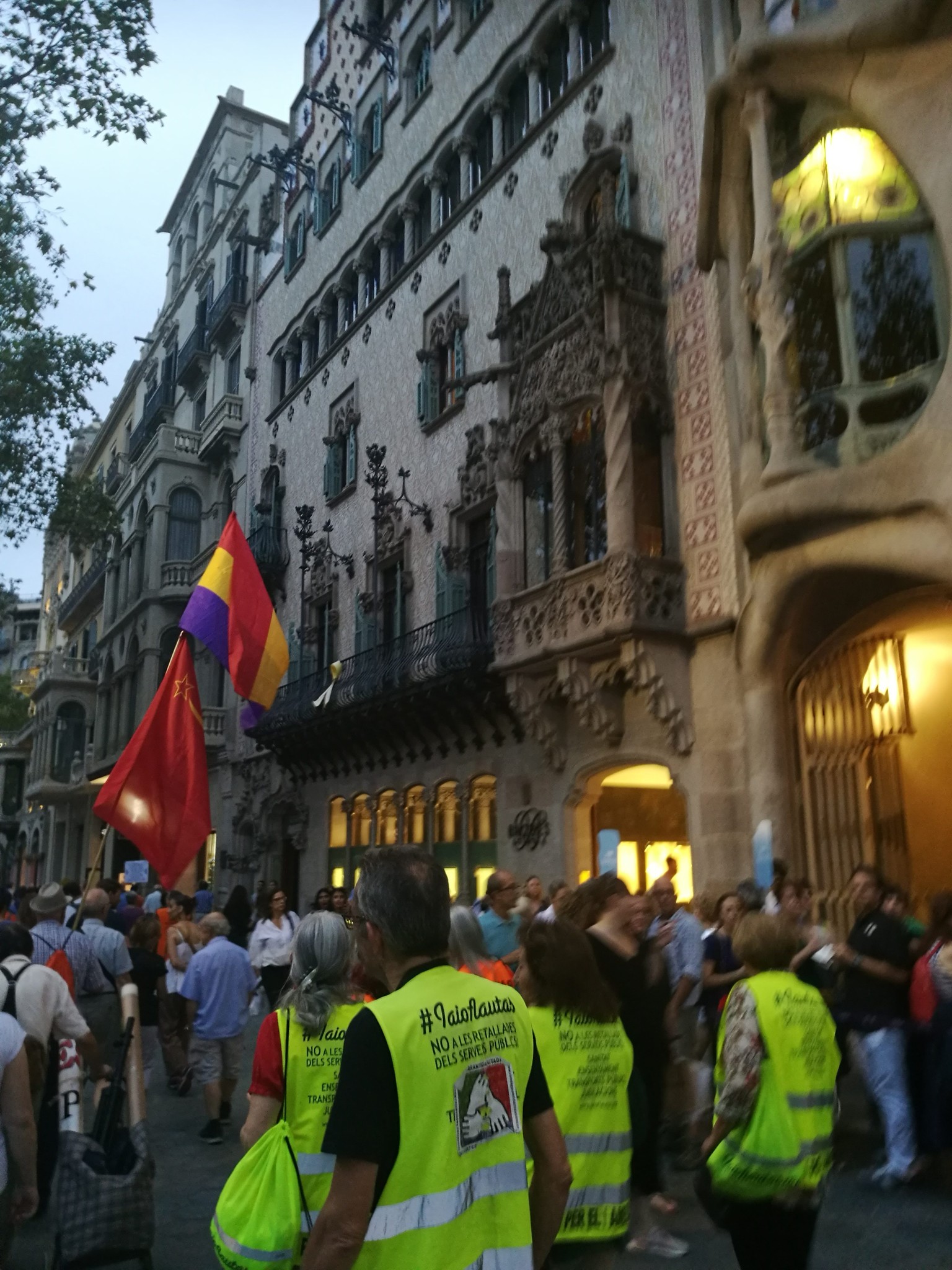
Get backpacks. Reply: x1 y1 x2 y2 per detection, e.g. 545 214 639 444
208 1004 316 1270
27 930 74 1002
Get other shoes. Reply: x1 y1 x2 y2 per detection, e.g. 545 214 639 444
198 1122 224 1143
219 1103 232 1124
628 1227 688 1256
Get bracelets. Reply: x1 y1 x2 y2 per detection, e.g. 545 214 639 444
850 951 863 968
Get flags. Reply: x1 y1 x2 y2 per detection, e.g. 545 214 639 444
177 510 289 711
100 640 214 896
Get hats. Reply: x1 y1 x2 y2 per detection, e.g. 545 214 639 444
29 882 73 913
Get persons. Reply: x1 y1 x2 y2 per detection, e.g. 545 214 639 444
308 857 952 1259
507 922 635 1270
224 878 300 1012
690 911 840 1270
0 920 115 1270
241 910 366 1270
0 876 259 1145
297 845 575 1270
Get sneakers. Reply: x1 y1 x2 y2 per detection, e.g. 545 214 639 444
169 1076 182 1090
178 1067 196 1096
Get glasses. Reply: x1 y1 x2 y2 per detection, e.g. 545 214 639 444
273 896 288 903
342 913 378 930
499 883 526 892
